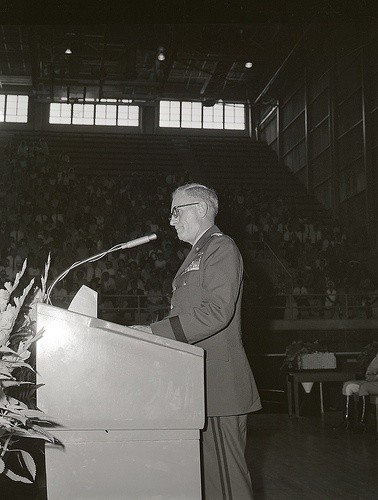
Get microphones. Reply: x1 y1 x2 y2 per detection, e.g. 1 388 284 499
47 231 158 311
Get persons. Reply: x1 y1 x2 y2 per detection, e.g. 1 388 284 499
132 183 261 499
0 136 377 318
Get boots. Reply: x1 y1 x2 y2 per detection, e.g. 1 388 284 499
337 394 354 429
350 394 370 436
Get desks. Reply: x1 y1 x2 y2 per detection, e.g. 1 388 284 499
282 368 356 417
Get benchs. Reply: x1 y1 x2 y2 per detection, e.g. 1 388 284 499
1 126 378 307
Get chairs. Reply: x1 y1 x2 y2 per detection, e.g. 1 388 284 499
342 353 378 422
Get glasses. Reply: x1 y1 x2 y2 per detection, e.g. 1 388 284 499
167 202 199 221
